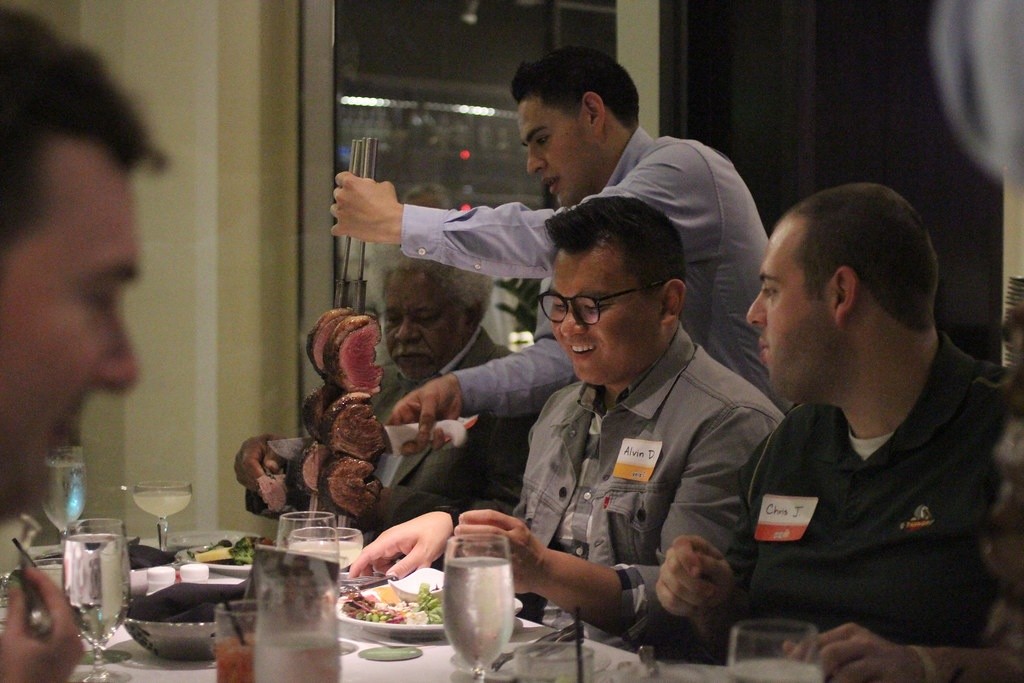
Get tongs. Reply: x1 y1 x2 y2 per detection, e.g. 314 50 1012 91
489 620 584 674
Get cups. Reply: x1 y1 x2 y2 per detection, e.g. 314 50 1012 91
291 526 340 565
277 511 337 550
519 643 593 683
337 527 364 570
215 599 255 683
727 621 825 683
255 601 340 683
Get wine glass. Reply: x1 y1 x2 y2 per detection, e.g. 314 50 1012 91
62 518 132 683
442 534 515 683
40 446 87 560
132 481 193 551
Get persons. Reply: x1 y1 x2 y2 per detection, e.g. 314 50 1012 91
348 195 784 662
330 47 796 458
0 1 169 683
656 183 1023 683
232 252 540 547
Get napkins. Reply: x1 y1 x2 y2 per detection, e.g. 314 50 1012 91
127 578 247 624
127 537 178 569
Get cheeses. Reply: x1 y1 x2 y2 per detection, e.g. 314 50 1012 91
195 547 234 562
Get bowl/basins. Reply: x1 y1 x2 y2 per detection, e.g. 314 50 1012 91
123 618 214 661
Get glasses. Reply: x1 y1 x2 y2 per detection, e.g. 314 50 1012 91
537 280 669 325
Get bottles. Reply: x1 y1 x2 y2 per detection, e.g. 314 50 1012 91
146 566 175 596
180 564 209 585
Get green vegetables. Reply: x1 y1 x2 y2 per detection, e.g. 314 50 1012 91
206 537 257 565
416 581 444 624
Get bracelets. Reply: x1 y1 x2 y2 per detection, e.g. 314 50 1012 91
909 644 935 682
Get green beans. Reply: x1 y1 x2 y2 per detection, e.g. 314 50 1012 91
356 612 389 622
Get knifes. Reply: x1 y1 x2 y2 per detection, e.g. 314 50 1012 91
266 418 467 463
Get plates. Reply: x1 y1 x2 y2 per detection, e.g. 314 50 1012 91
168 530 262 547
175 544 279 575
337 568 523 638
450 642 612 681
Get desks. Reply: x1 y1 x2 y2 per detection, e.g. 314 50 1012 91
0 530 669 683
